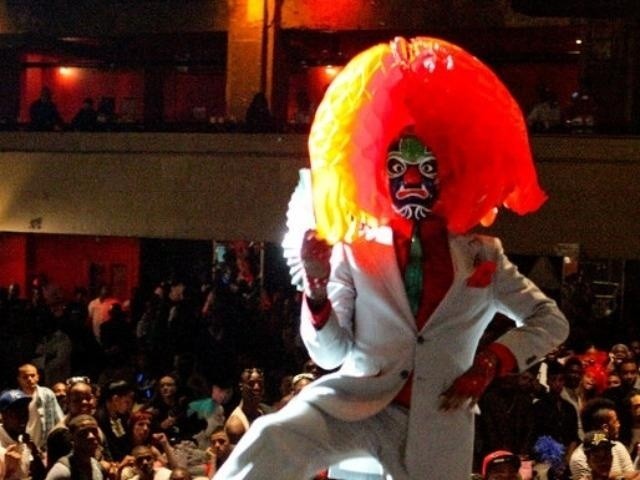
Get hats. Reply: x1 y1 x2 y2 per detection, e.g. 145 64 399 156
583 430 616 452
483 451 521 476
0 389 32 412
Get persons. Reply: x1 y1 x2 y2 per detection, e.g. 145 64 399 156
1 237 640 478
2 85 141 131
164 91 282 133
511 85 638 136
219 36 571 480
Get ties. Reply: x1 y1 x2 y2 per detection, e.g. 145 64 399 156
403 234 423 316
